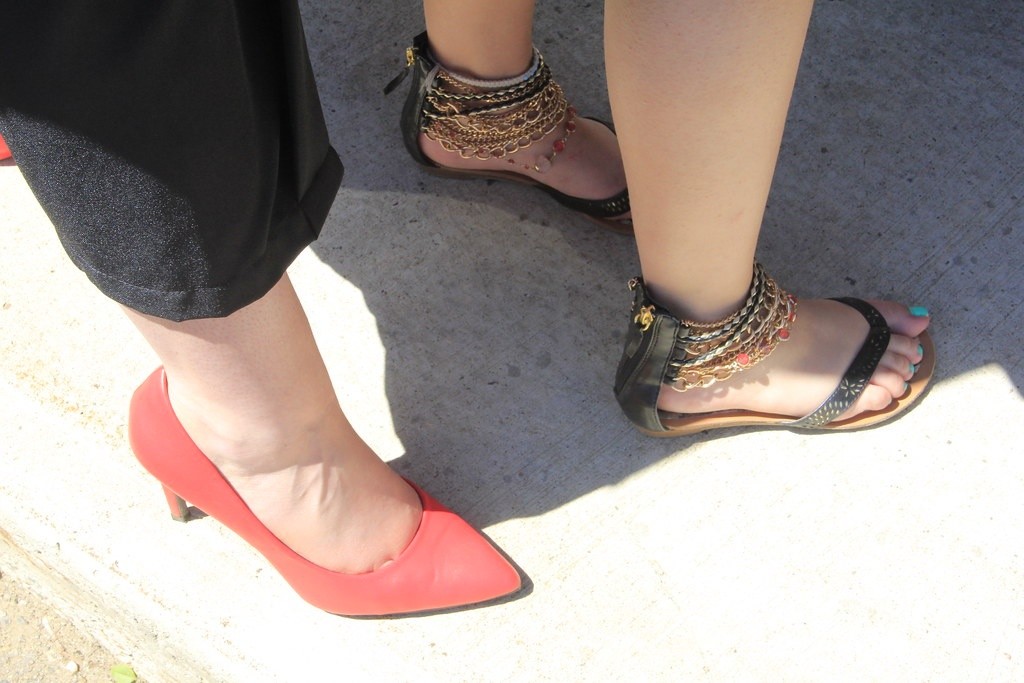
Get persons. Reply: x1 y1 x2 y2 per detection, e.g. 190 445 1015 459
383 0 936 438
0 0 522 616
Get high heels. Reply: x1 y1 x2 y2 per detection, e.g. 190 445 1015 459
128 365 524 616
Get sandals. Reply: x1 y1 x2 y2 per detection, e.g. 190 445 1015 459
613 262 938 439
383 30 634 237
